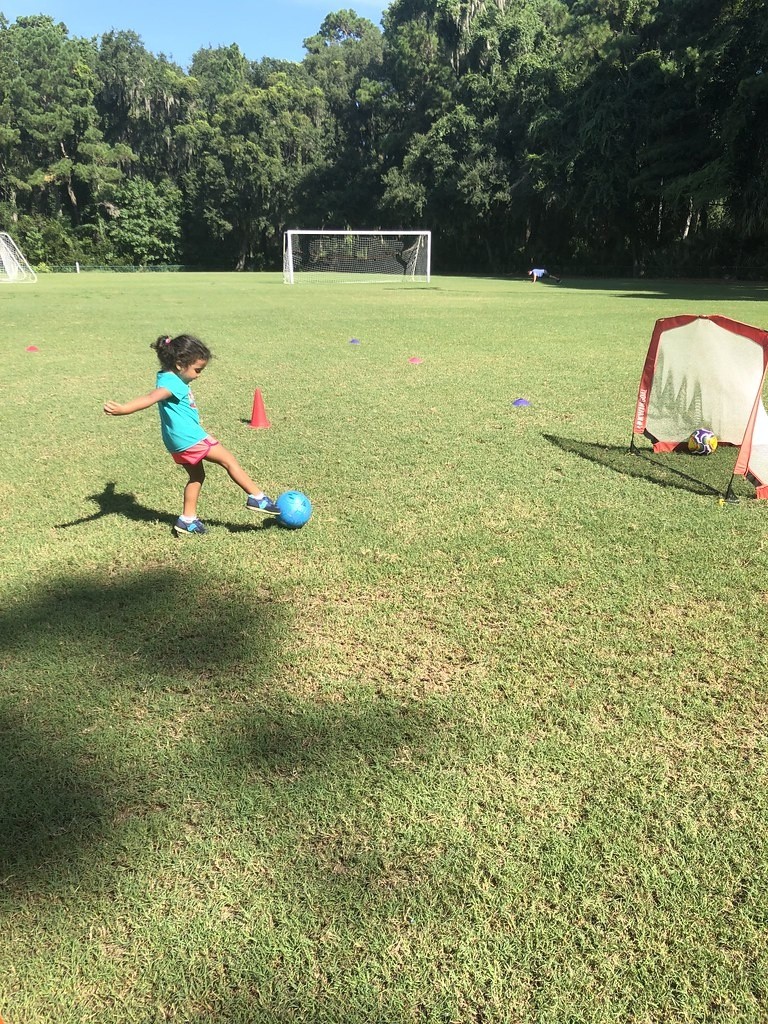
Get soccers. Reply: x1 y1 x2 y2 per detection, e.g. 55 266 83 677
687 428 719 456
274 490 314 529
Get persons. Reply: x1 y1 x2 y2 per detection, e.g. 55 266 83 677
527 269 561 283
104 334 281 534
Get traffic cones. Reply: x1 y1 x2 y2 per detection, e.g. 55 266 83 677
247 387 272 429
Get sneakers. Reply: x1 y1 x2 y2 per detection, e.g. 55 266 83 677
173 519 209 534
246 496 281 515
